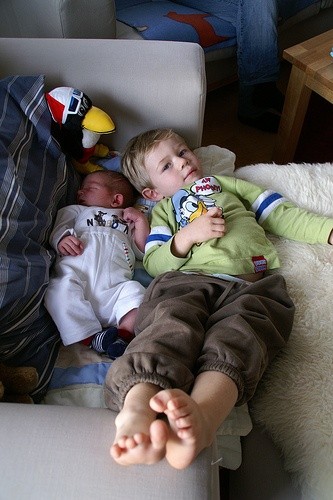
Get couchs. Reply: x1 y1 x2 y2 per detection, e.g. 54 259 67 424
0 38 252 500
0 0 333 90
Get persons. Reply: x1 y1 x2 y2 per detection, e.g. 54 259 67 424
103 126 333 470
42 168 150 357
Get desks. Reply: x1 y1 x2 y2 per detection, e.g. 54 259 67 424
276 30 333 162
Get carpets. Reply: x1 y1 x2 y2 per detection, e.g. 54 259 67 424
235 162 333 500
